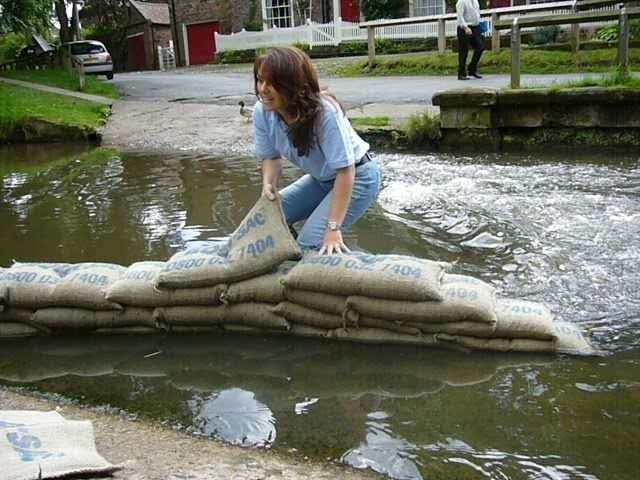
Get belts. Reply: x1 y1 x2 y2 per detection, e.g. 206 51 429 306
355 152 372 168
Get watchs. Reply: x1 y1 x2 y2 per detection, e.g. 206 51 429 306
326 223 339 231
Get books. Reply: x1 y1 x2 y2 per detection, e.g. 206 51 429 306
480 21 487 33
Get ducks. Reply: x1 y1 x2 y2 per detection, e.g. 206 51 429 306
238 100 253 122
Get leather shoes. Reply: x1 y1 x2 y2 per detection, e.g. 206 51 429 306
458 76 470 79
468 71 482 78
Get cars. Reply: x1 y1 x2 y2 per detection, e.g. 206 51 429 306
59 39 114 80
15 43 59 70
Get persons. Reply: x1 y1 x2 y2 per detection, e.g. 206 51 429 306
455 0 482 80
252 46 379 256
